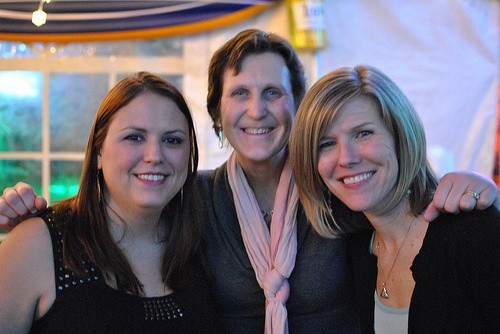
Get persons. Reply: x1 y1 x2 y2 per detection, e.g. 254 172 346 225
289 64 500 334
0 71 226 334
1 28 500 334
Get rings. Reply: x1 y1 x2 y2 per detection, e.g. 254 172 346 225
465 190 481 200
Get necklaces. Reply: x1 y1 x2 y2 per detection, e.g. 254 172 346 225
377 215 415 298
260 210 273 218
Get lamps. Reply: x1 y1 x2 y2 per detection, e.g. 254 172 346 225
32 0 51 27
